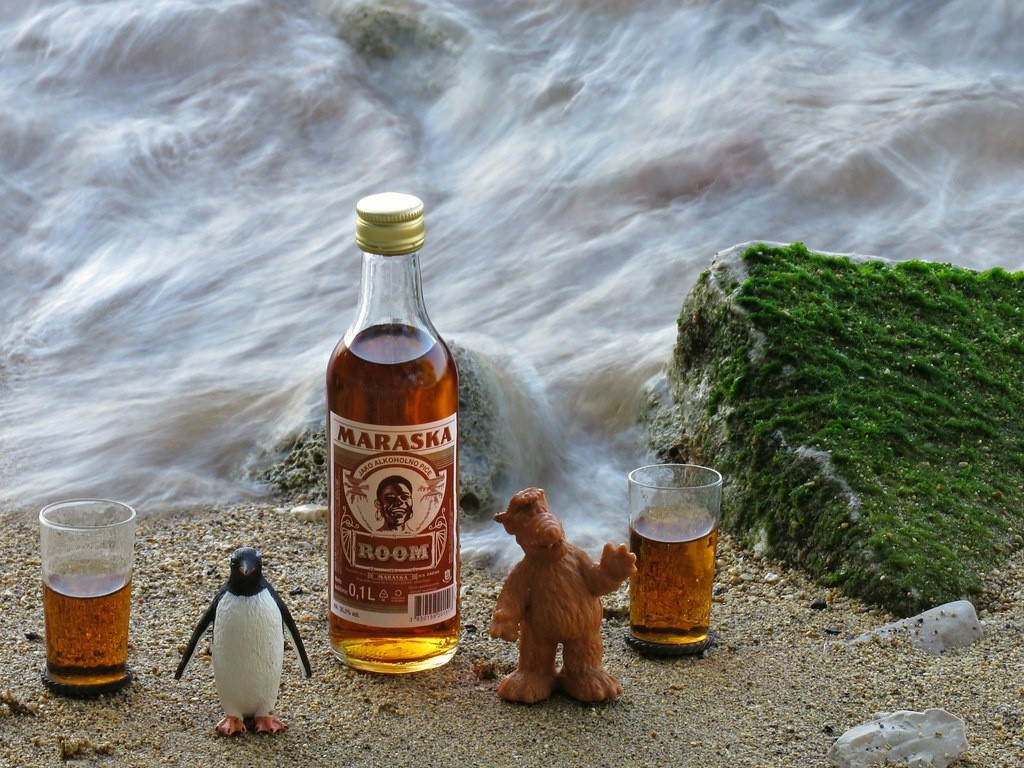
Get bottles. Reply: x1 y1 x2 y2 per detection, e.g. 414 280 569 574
321 191 464 674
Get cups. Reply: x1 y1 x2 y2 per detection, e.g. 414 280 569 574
38 496 136 685
627 463 724 647
